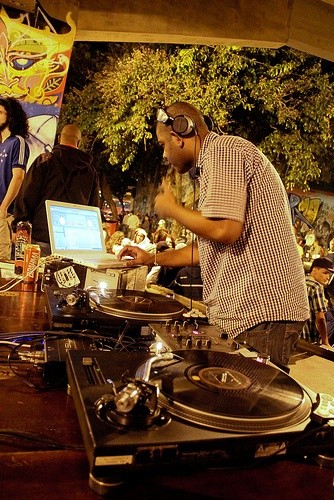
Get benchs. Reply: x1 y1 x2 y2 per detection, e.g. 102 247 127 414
151 284 209 318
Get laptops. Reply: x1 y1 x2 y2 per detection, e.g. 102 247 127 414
45 200 143 269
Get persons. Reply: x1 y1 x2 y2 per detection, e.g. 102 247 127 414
13 124 101 260
294 234 329 258
0 97 31 260
108 210 186 265
118 100 311 369
297 257 334 351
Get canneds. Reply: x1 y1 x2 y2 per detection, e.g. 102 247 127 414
23 244 41 283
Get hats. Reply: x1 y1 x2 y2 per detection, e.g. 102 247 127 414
312 258 334 273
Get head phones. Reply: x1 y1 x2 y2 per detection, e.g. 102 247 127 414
156 108 195 137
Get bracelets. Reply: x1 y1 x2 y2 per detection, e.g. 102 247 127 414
153 252 158 266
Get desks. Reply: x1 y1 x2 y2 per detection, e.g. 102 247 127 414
0 238 334 500
